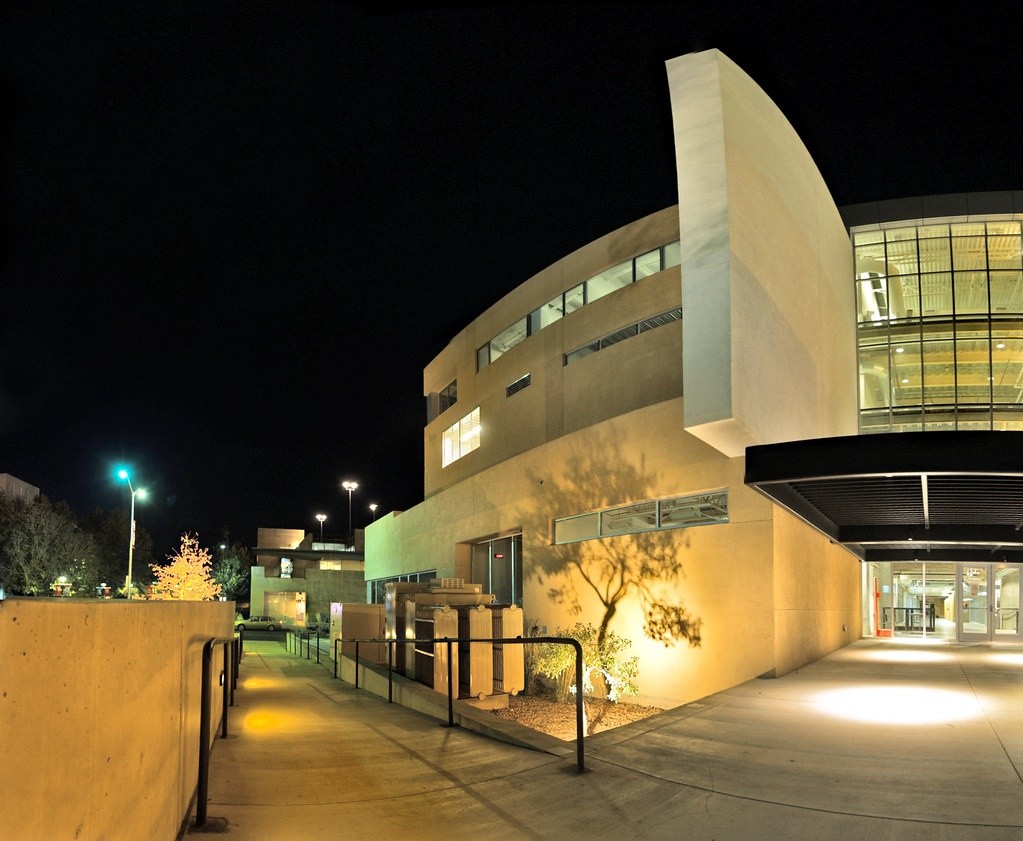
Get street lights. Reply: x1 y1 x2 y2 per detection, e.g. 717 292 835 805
118 470 146 600
341 480 358 551
316 513 327 543
220 544 226 603
